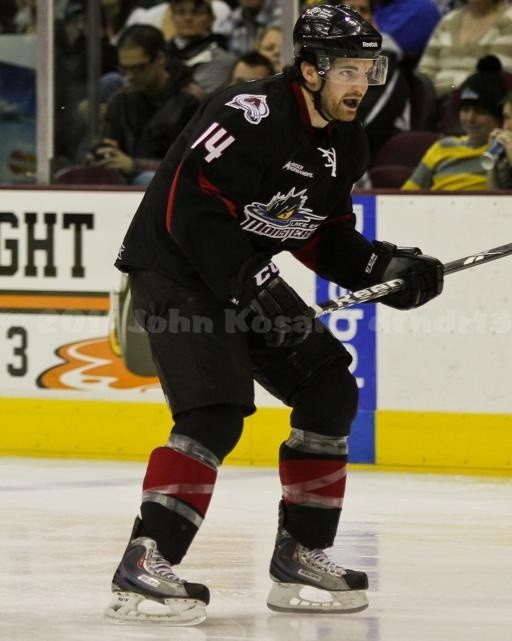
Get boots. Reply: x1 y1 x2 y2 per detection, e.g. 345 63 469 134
269 500 369 591
111 517 209 606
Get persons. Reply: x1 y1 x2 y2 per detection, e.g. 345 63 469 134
107 5 447 607
1 0 511 199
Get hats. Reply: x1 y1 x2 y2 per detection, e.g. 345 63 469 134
456 55 504 126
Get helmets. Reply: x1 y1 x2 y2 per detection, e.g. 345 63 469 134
293 4 389 87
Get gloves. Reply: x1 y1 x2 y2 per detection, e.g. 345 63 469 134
359 240 444 311
236 261 314 350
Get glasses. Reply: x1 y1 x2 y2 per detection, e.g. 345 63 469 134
116 62 149 75
